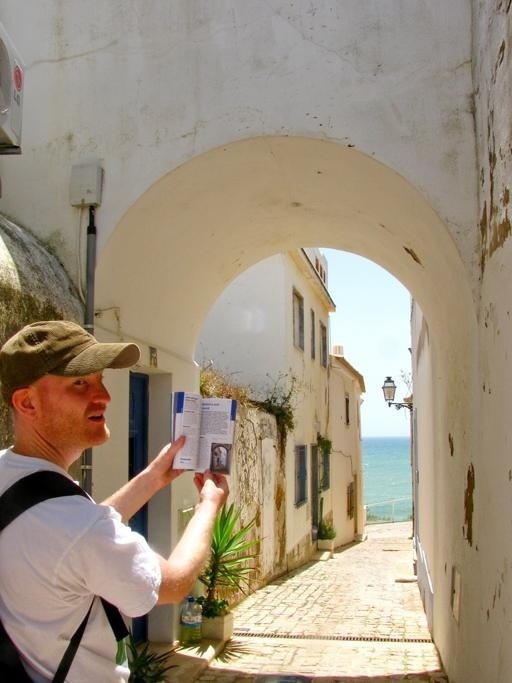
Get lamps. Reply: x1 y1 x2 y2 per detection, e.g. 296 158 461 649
382 377 413 409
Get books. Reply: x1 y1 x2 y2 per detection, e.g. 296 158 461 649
169 391 237 475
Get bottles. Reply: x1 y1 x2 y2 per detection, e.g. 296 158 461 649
181 595 203 643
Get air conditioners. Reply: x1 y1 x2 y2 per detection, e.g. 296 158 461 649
0 22 25 148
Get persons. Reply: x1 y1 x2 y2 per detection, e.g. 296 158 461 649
0 319 230 683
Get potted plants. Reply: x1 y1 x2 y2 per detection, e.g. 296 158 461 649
317 519 337 551
194 499 268 640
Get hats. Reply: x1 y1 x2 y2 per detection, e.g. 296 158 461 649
0 319 141 412
